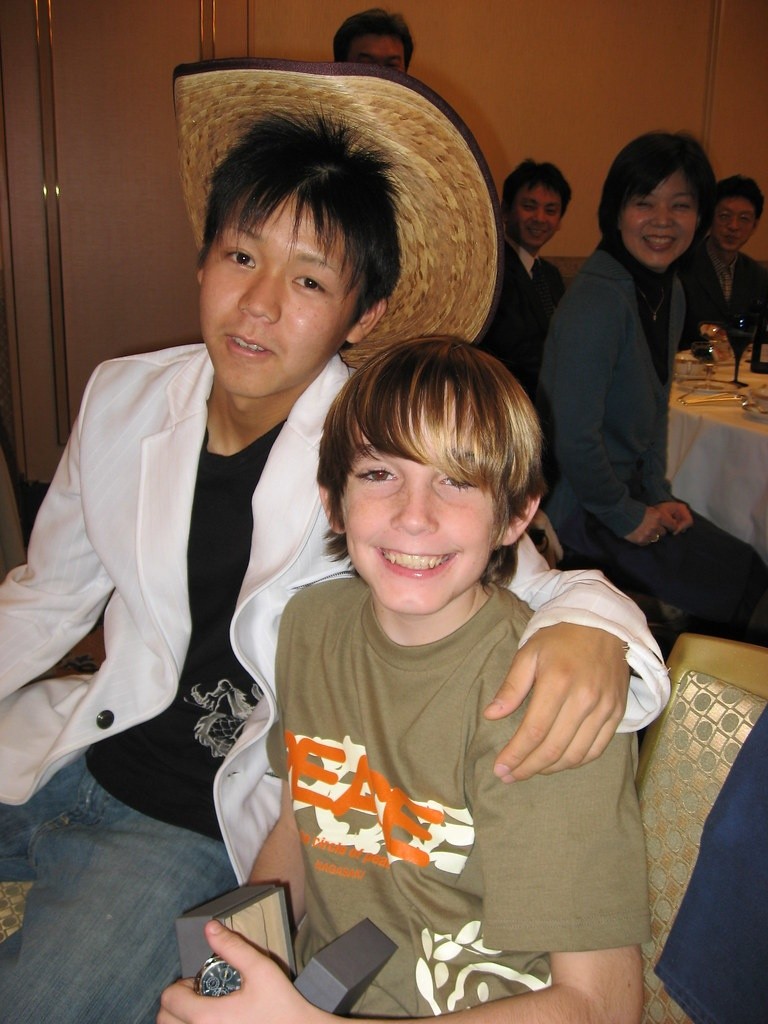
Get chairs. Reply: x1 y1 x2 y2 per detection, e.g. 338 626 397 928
632 634 768 1024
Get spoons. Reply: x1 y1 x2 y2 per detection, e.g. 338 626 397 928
678 387 746 405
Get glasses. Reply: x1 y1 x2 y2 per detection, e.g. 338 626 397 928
720 211 753 223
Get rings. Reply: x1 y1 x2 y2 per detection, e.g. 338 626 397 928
651 534 659 543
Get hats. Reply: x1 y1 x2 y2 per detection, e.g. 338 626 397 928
172 56 506 372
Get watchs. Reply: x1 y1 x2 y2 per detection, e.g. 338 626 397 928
194 953 241 997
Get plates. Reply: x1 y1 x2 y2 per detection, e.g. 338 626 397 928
672 374 705 378
682 379 736 392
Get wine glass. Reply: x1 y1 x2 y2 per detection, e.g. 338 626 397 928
727 312 758 389
692 321 732 395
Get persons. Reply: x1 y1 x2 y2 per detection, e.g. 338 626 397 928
1 102 671 1024
332 8 767 641
156 335 650 1024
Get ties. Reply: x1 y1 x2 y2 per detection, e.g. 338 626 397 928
531 258 554 320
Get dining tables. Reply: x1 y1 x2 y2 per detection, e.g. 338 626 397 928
667 345 768 565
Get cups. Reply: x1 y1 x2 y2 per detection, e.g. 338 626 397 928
674 352 704 374
